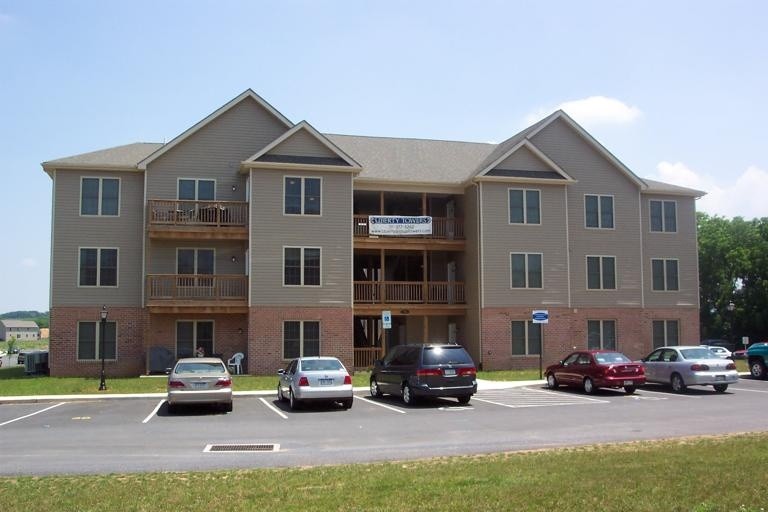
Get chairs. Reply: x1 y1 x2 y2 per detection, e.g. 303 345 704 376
227 352 245 375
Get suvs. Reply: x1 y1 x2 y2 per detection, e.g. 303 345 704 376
747 342 767 379
17 352 27 364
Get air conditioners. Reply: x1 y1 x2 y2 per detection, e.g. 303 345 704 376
23 351 48 375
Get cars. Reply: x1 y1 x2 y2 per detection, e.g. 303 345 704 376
633 344 739 394
8 347 20 354
700 345 732 360
733 342 767 358
277 353 353 410
0 356 3 367
163 356 234 412
544 347 647 397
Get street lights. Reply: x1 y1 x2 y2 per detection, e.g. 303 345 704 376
97 305 109 391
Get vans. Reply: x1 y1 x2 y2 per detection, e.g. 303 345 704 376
368 342 478 407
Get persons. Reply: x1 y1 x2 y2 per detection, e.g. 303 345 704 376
196 346 203 358
